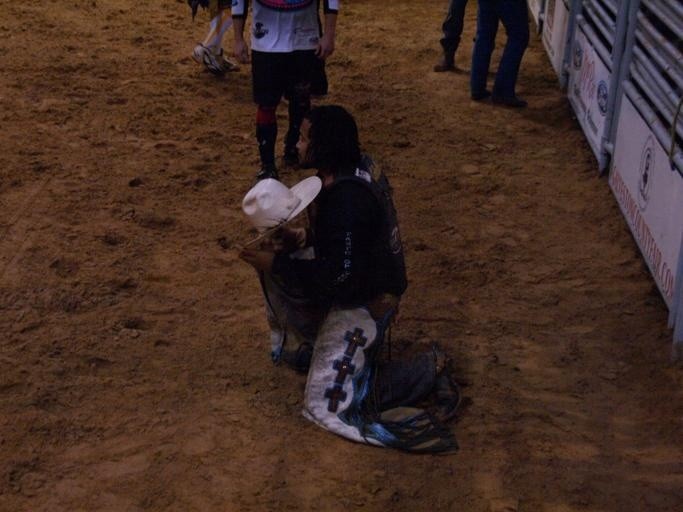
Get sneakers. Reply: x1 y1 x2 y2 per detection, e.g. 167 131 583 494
283 147 313 169
190 43 240 77
471 90 492 101
251 168 280 188
433 55 454 72
492 97 528 108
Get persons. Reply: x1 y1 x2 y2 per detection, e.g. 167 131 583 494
176 0 249 79
469 0 531 108
432 0 467 72
229 0 338 181
232 102 457 451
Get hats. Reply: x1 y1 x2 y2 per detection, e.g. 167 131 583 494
241 176 323 247
